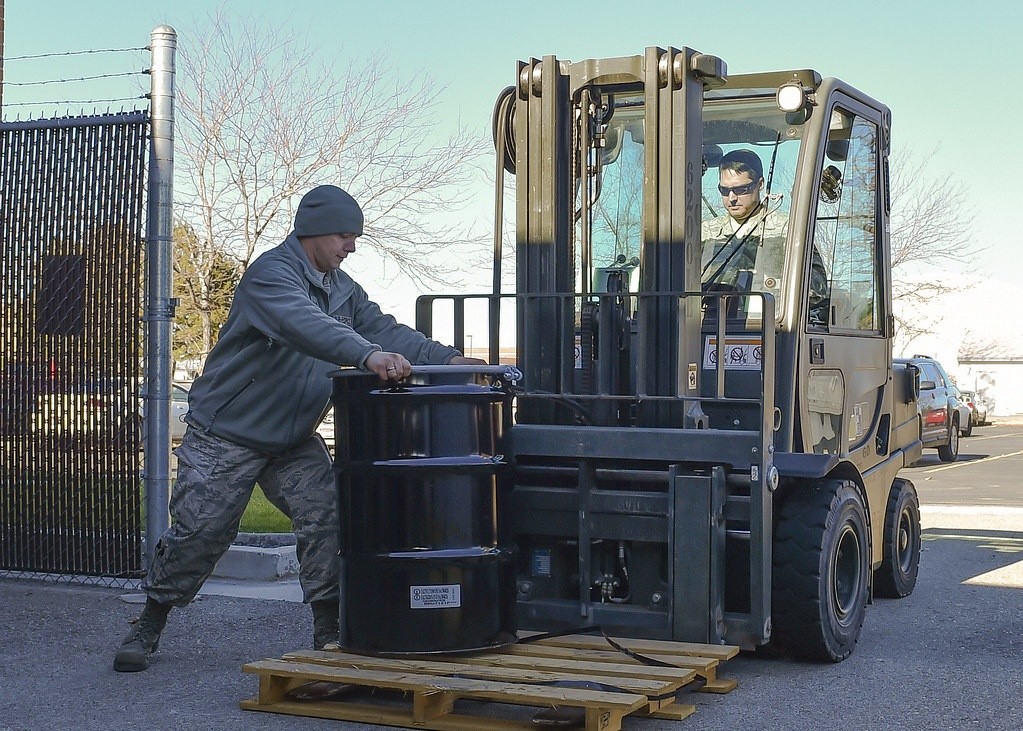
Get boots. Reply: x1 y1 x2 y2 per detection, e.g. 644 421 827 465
310 601 341 650
114 592 172 672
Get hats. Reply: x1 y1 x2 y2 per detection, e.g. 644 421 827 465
294 184 364 237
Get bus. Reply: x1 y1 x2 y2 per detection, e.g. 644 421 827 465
892 355 960 462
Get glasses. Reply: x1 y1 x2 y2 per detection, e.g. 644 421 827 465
718 179 759 197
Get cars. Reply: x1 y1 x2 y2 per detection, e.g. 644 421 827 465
33 375 189 448
954 386 988 436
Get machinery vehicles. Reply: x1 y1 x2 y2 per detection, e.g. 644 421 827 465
238 45 923 731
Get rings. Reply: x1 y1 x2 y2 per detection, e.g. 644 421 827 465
387 366 395 373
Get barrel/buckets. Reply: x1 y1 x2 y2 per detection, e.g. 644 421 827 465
326 365 519 655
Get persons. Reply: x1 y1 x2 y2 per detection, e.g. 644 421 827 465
114 185 489 672
698 150 833 333
